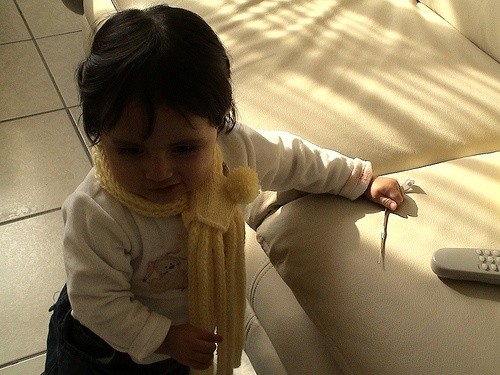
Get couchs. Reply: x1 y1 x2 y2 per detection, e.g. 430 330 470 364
60 0 500 375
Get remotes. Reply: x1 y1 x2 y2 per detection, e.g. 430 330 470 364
431 248 500 285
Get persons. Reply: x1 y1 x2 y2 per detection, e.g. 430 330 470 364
39 2 405 375
